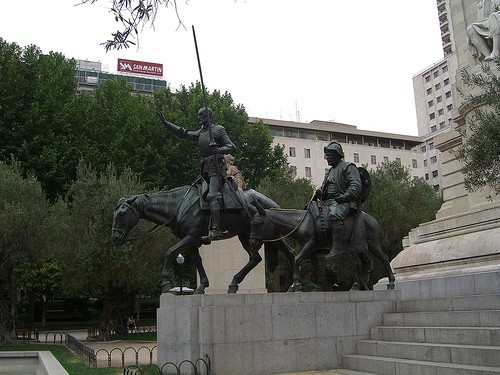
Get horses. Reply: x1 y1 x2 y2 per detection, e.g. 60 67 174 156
240 195 396 294
111 184 285 294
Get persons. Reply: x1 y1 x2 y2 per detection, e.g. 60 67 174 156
155 106 236 238
466 0 500 61
223 154 248 191
316 141 363 259
128 315 135 333
